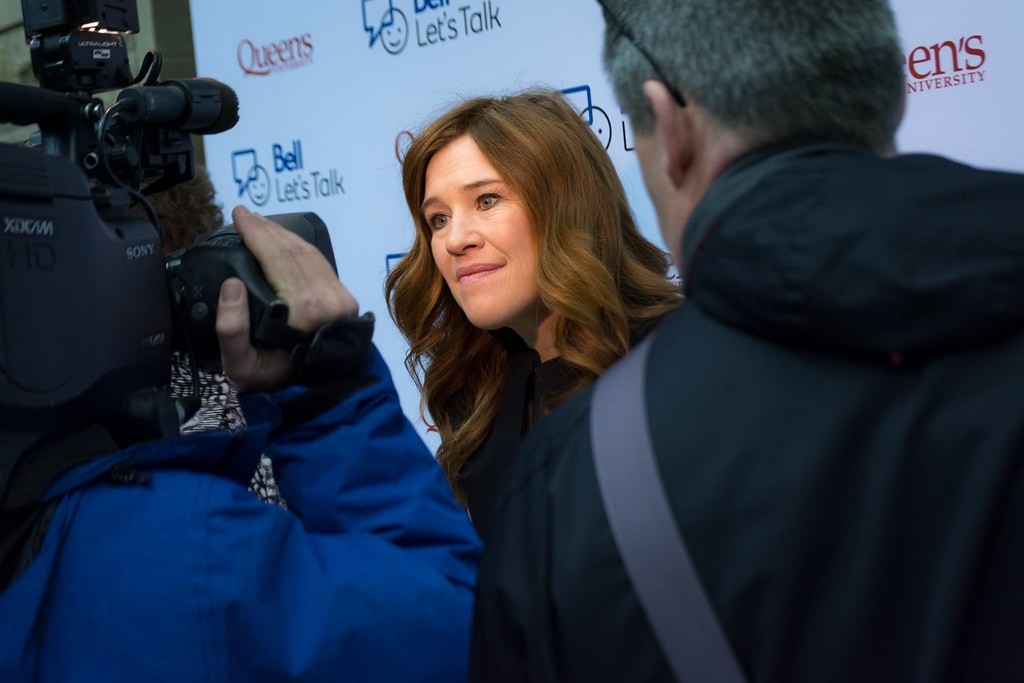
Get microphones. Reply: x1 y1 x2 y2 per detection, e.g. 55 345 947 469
115 77 240 135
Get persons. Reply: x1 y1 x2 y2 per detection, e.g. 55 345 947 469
378 82 685 498
469 0 1024 683
0 140 482 683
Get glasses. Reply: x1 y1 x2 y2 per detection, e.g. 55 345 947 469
598 0 686 108
